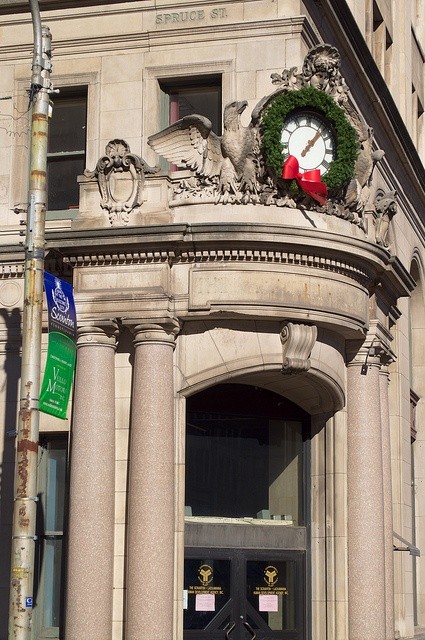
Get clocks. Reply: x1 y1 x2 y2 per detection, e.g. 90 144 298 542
278 107 337 182
